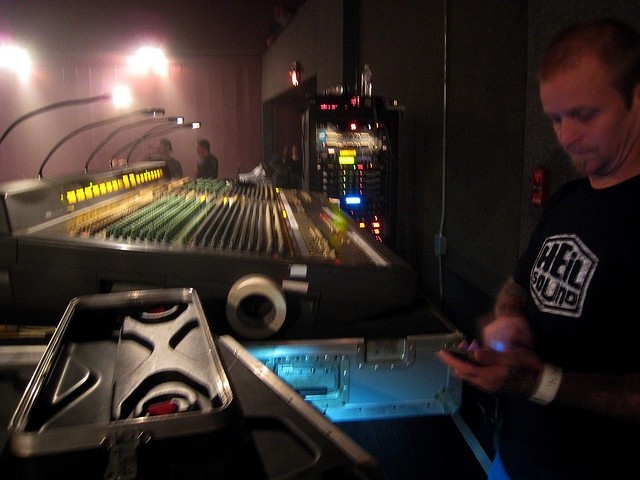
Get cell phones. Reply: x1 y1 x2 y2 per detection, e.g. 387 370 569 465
443 347 494 367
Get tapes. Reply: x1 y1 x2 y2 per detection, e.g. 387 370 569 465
225 273 287 341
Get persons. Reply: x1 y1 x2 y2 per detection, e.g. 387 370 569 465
195 138 218 180
158 138 183 180
435 21 639 480
